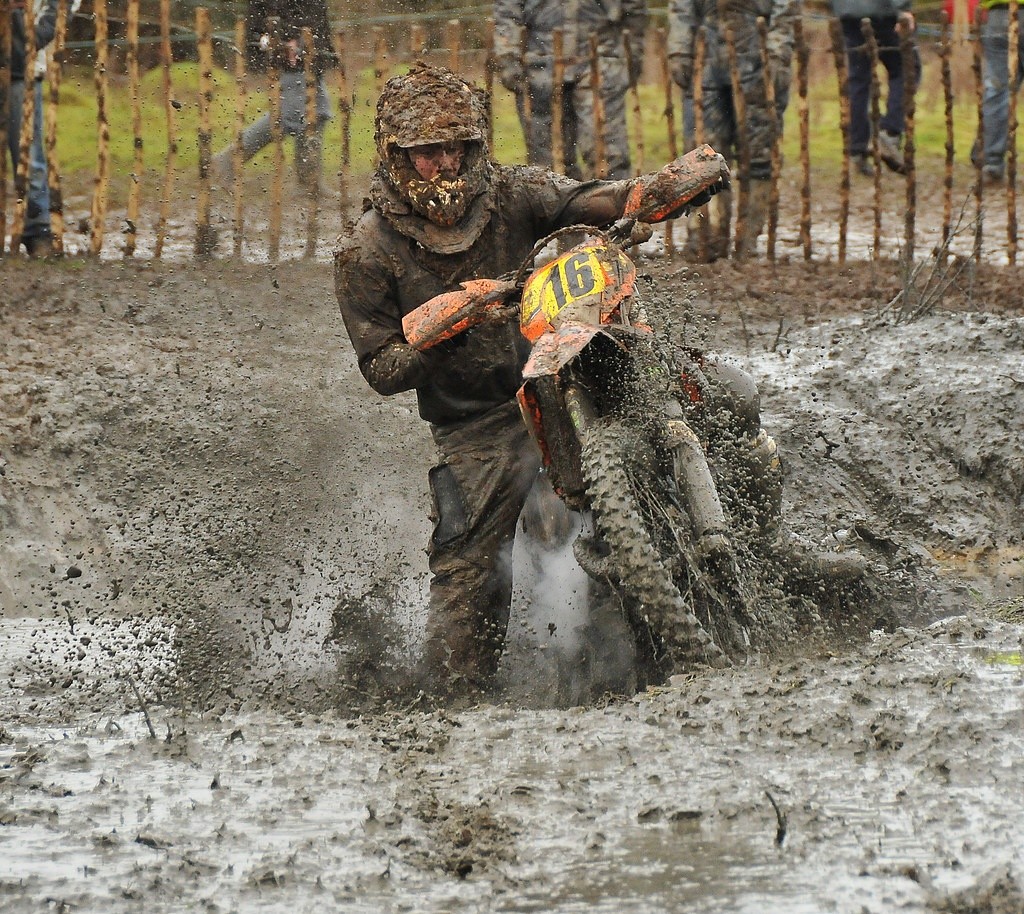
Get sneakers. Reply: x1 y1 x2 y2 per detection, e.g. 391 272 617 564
850 155 877 179
865 128 912 176
757 526 865 595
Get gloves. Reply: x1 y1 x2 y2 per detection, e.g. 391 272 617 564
420 330 470 357
666 55 695 90
497 56 527 96
764 59 792 93
690 151 732 207
627 55 643 88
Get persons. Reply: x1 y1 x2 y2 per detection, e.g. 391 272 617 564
0 0 1023 266
334 58 871 711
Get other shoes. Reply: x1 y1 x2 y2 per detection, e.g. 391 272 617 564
26 236 61 262
209 148 250 203
980 164 1011 188
289 180 341 205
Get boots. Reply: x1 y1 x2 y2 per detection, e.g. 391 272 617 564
704 179 776 263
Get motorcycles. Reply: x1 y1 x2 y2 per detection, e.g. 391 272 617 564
400 144 761 688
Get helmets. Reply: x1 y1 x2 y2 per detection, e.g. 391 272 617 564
372 60 494 228
